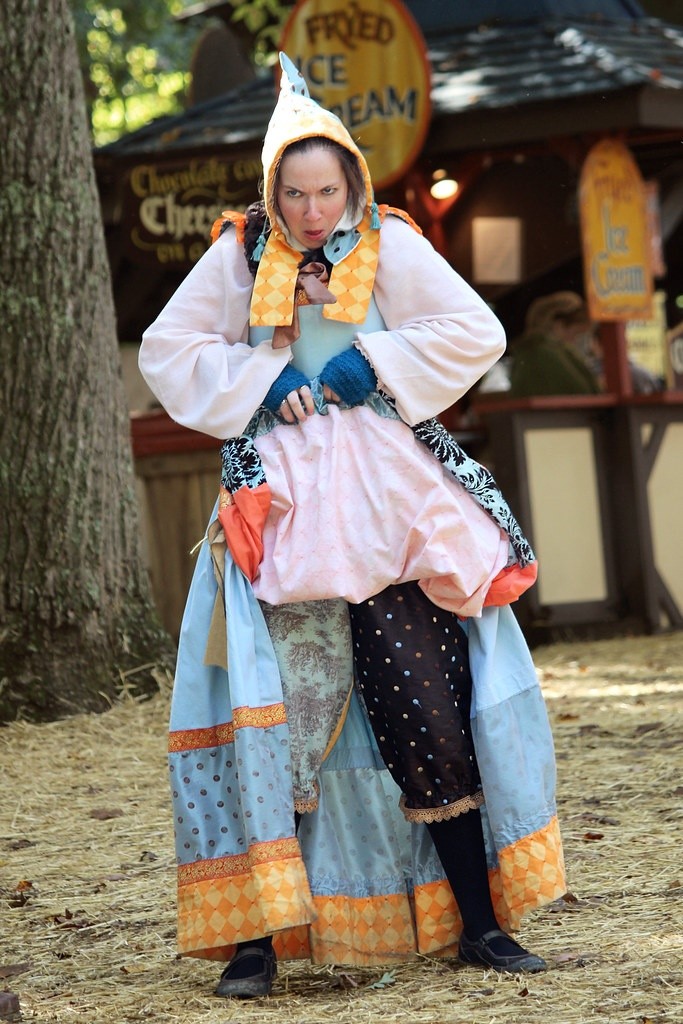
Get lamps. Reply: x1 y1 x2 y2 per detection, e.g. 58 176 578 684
429 165 458 198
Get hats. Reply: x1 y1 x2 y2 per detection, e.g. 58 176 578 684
249 52 382 327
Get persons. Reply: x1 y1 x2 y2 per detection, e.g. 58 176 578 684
136 52 566 999
509 292 658 398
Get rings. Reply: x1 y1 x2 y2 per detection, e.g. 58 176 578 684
281 399 287 404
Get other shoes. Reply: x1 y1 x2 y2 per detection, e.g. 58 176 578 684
216 945 277 997
458 930 547 974
